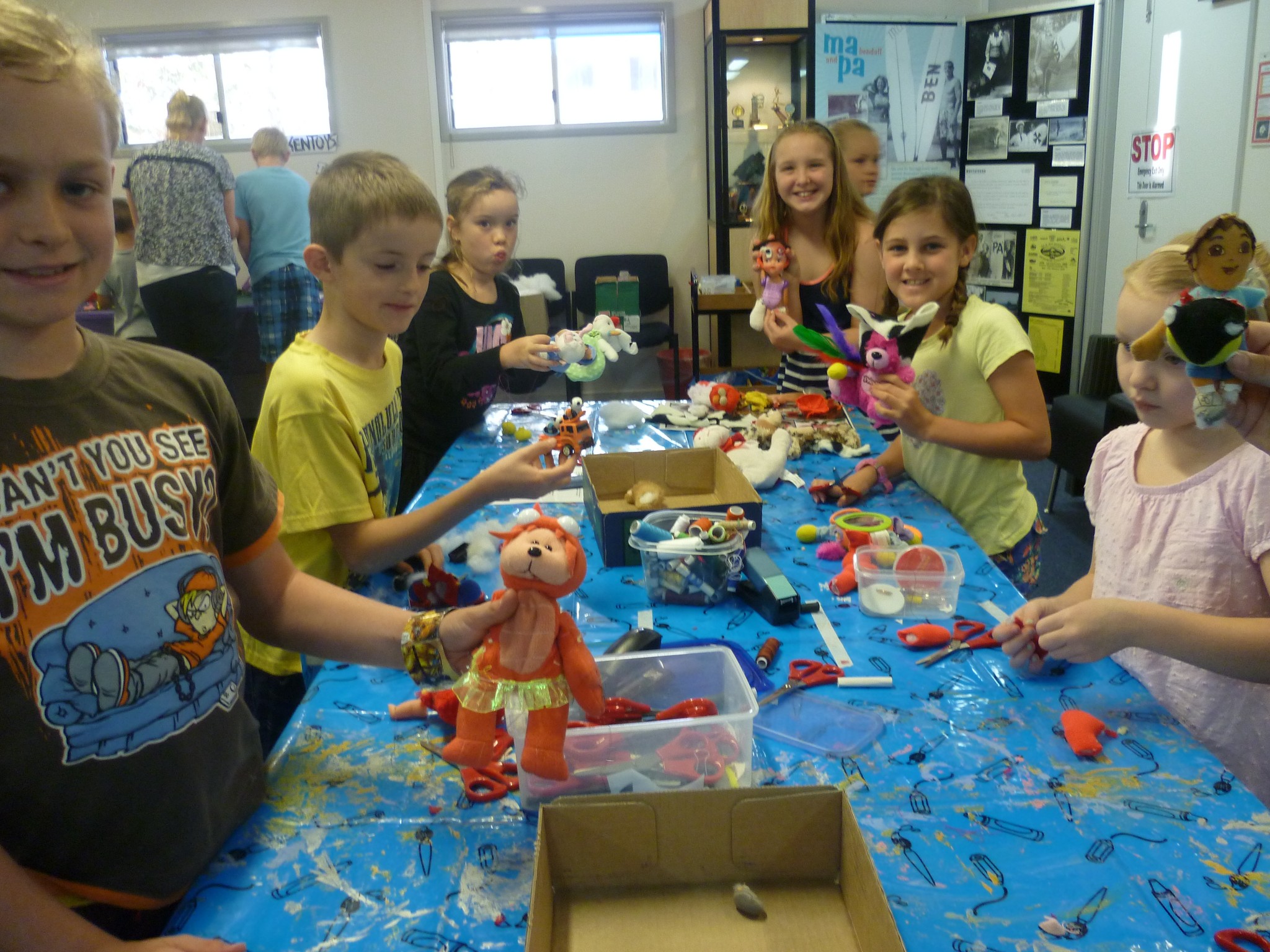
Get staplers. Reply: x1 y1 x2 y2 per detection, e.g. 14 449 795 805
733 547 800 626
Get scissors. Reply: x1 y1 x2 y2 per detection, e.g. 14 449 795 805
529 697 738 796
758 660 845 707
419 740 524 803
490 728 515 762
915 620 1009 667
808 467 863 500
511 404 557 421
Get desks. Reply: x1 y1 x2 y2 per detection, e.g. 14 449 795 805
166 397 1269 952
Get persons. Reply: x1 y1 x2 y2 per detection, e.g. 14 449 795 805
989 229 1270 815
825 15 1085 292
227 149 582 746
825 120 882 202
96 193 162 345
393 164 561 504
121 88 262 447
1219 319 1270 454
0 2 522 871
233 126 322 381
808 175 1052 599
750 119 888 401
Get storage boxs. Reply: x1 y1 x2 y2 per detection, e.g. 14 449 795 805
700 274 736 296
853 543 967 621
501 644 760 813
519 292 550 337
575 445 764 569
594 272 641 334
627 509 751 607
524 785 907 952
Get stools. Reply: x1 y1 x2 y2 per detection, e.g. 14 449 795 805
1043 390 1108 513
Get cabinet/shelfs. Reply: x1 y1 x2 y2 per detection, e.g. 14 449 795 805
688 268 780 396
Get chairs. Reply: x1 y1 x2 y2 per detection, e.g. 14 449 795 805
570 254 682 401
507 258 573 402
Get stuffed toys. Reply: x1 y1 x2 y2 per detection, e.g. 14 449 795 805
750 239 791 331
438 497 591 778
1130 215 1268 430
682 379 869 489
791 298 937 427
535 314 639 382
